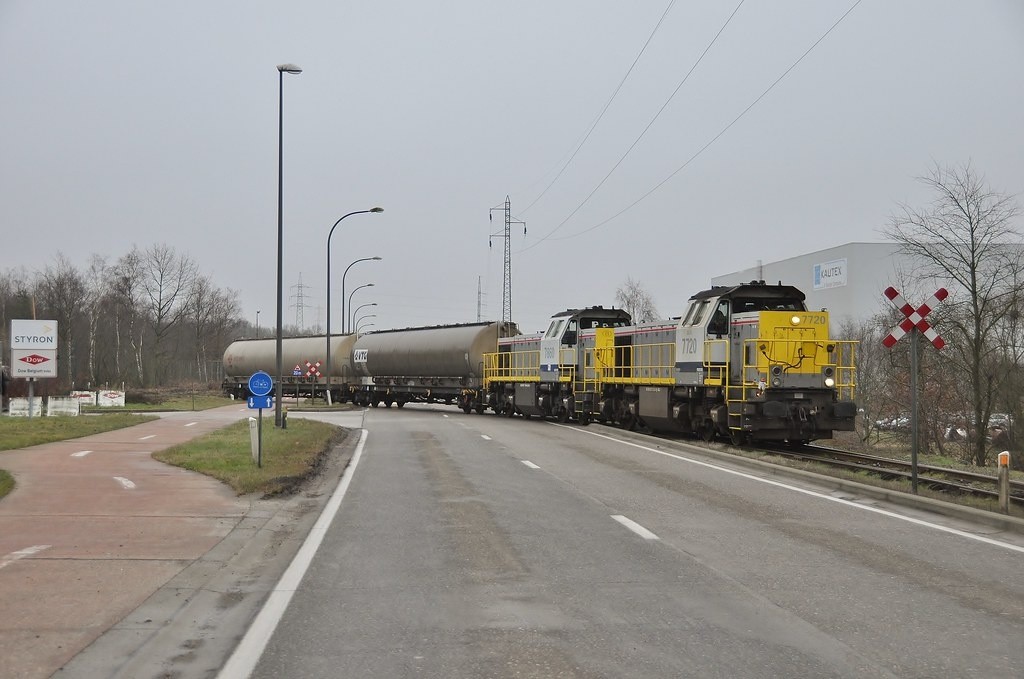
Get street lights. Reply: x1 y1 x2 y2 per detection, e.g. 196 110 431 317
348 283 375 334
326 206 384 407
353 303 378 334
342 256 382 336
274 62 305 432
355 314 376 342
256 310 261 340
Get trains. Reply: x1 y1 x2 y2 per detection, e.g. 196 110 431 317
220 278 859 450
